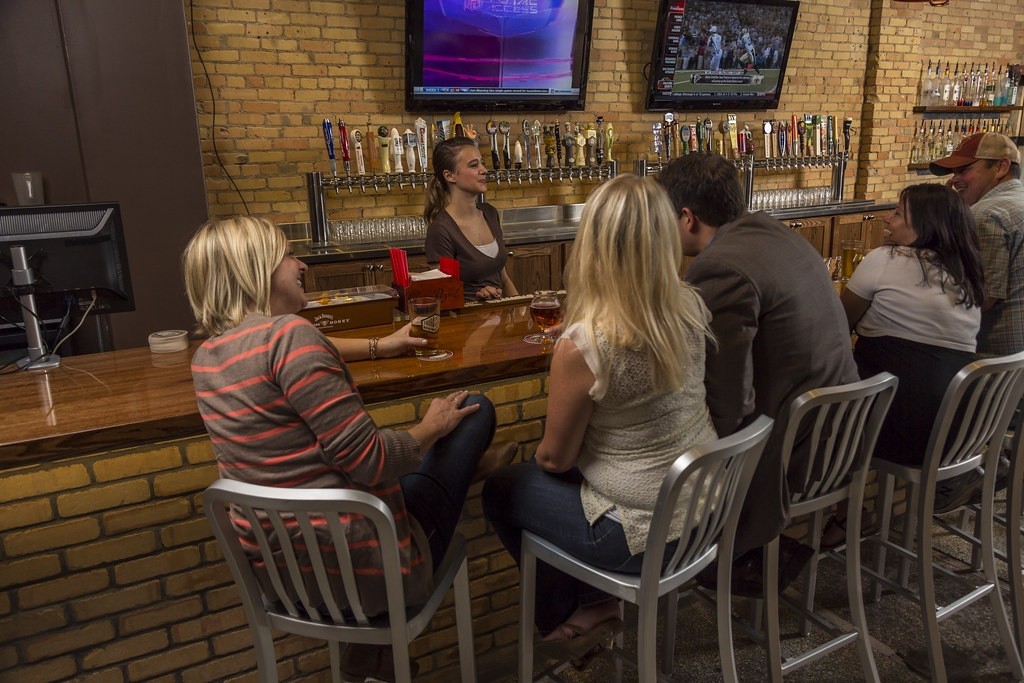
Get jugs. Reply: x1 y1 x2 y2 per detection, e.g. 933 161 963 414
10 169 42 209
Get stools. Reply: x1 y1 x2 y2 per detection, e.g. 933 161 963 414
206 479 472 682
660 368 898 682
798 348 1024 682
898 401 1024 659
518 413 779 683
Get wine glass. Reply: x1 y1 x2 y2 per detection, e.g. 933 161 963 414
529 293 560 352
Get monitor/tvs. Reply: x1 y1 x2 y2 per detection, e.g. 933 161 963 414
0 200 136 371
403 0 595 110
644 0 801 111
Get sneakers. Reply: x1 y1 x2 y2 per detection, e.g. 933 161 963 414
933 466 985 514
995 455 1013 490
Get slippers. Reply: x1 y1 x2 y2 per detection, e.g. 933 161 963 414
570 639 605 672
821 515 881 550
534 616 623 661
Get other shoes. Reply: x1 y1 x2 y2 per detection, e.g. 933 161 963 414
742 536 816 598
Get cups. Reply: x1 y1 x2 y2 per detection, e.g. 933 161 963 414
747 186 834 210
408 297 440 355
840 238 866 281
326 211 439 244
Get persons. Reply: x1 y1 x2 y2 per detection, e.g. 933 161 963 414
929 132 1024 514
821 184 984 552
423 137 526 317
678 5 788 74
464 124 477 140
183 214 518 682
657 151 863 599
483 172 728 661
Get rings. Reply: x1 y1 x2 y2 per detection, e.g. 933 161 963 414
455 399 459 405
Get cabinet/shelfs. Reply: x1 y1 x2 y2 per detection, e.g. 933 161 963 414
779 216 832 258
831 209 897 254
908 103 1024 170
503 244 561 296
299 252 430 293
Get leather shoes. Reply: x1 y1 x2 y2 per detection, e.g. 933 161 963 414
340 641 420 683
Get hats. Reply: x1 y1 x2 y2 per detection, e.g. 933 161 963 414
928 132 1020 175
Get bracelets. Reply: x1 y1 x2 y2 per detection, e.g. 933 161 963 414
369 337 379 359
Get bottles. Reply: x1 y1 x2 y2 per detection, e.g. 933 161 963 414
909 60 1018 165
